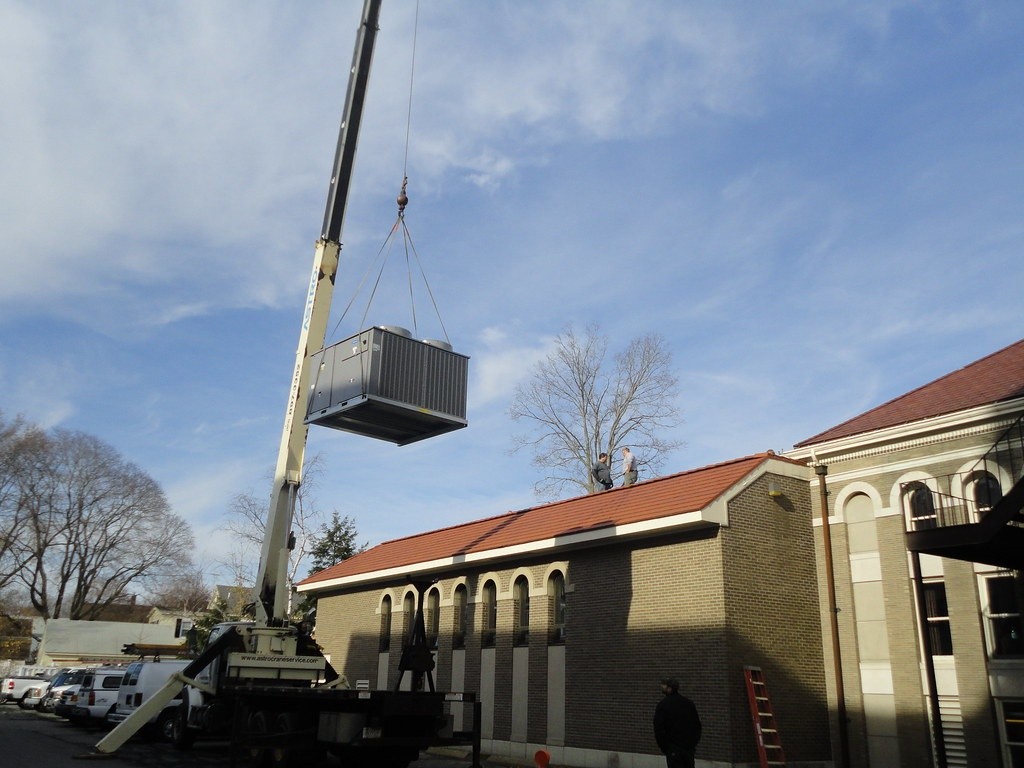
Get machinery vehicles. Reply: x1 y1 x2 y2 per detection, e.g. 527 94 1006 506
88 4 483 768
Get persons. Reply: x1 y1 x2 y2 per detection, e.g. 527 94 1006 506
652 675 702 768
593 452 615 492
618 448 638 486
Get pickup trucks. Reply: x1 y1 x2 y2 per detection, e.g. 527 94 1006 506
0 667 62 709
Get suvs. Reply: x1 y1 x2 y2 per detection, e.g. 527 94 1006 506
68 663 130 731
25 666 86 721
107 654 205 745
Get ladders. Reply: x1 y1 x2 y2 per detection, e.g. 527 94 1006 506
743 665 787 768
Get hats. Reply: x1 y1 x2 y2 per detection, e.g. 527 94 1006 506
661 678 679 689
